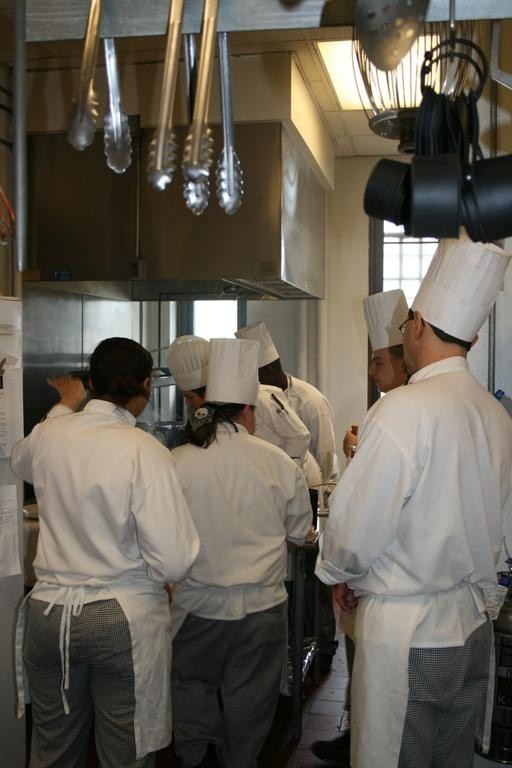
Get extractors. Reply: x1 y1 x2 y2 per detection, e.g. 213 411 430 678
20 118 328 304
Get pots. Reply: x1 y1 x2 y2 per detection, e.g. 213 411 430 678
361 85 512 243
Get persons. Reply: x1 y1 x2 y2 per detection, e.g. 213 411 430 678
3 332 202 767
311 305 511 768
158 400 314 768
308 344 411 768
181 383 338 674
257 357 341 497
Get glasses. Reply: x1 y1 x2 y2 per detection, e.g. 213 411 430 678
399 313 426 333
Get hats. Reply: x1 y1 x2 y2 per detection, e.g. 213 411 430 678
410 236 511 342
234 322 279 368
363 289 408 350
168 335 207 392
204 339 259 405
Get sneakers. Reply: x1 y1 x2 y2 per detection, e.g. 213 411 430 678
311 729 349 765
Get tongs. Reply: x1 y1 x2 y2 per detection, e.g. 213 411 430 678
66 0 135 177
142 0 212 218
181 0 250 217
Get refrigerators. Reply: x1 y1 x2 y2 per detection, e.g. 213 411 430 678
0 293 28 768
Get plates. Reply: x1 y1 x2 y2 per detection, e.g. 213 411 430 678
22 503 39 520
